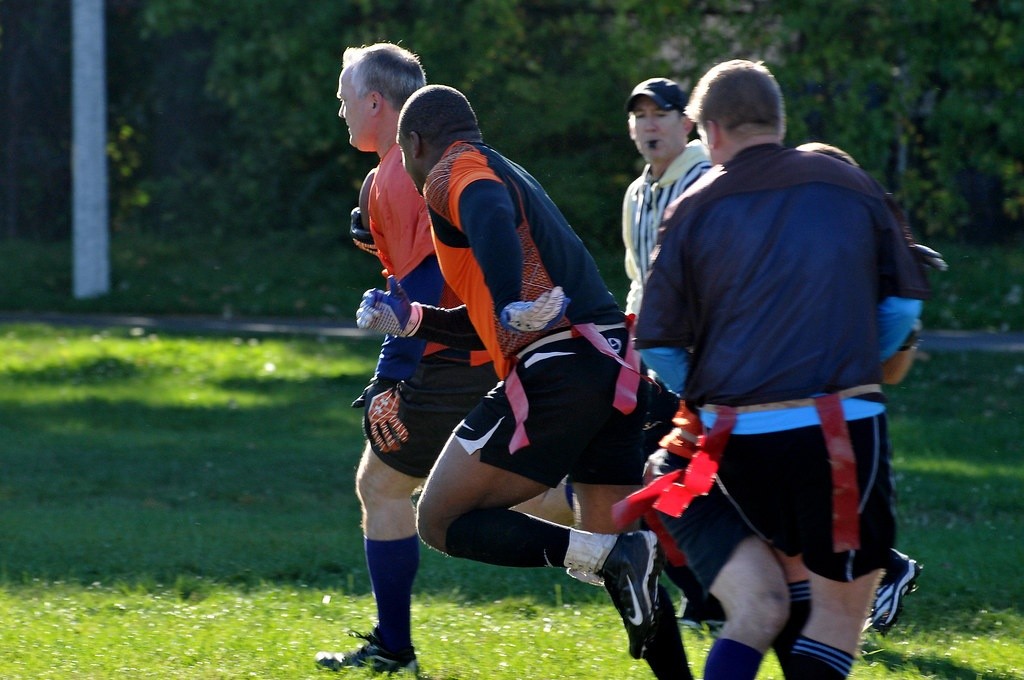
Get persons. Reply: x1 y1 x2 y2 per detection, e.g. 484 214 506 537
316 42 633 678
797 141 921 637
356 83 695 680
621 77 728 631
632 58 926 680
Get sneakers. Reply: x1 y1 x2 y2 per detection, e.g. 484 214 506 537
596 531 658 659
315 625 419 679
871 559 922 637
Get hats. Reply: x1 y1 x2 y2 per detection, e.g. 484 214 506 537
626 78 687 115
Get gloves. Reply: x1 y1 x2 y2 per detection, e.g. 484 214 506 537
500 286 570 334
907 236 949 282
356 276 419 337
351 381 409 454
641 446 688 487
